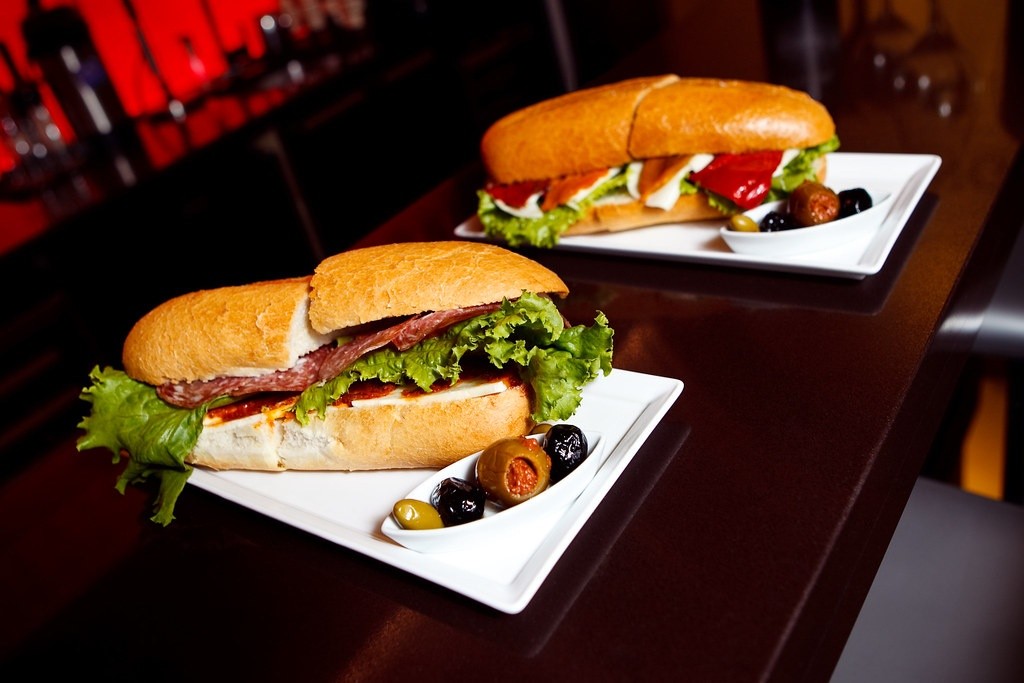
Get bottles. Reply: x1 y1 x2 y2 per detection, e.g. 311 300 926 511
758 0 853 124
0 0 152 191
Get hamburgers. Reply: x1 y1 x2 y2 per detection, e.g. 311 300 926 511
475 75 840 250
78 241 614 527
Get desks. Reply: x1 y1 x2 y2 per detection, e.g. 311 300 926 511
0 108 1024 683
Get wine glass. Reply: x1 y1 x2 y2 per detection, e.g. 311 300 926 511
858 0 974 167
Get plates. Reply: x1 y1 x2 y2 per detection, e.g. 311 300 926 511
719 185 903 254
187 366 684 614
452 151 943 276
380 432 605 553
